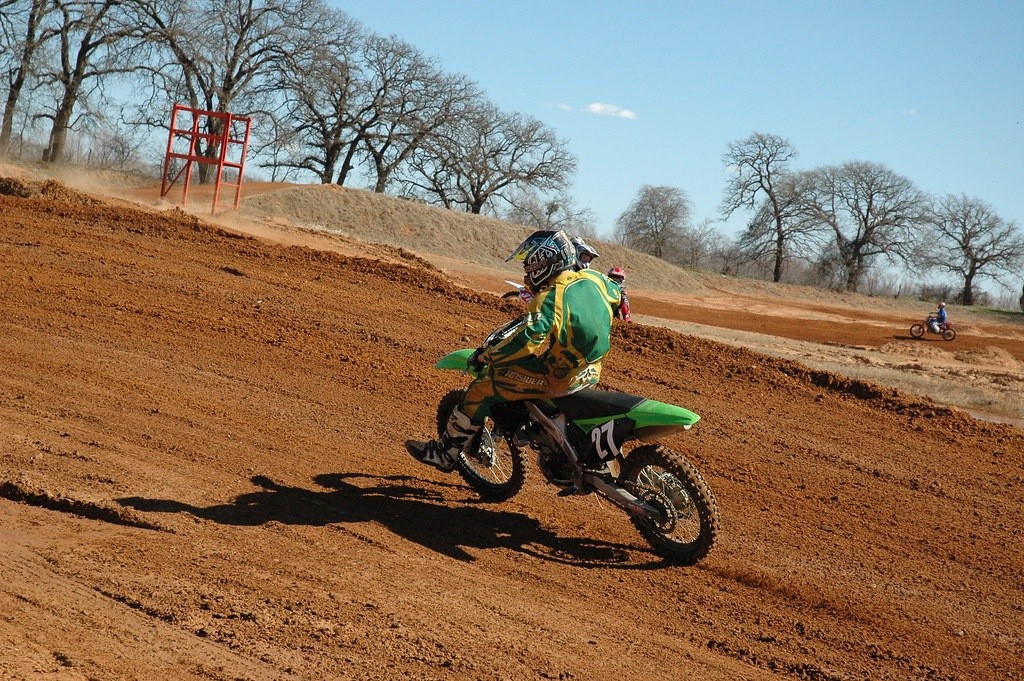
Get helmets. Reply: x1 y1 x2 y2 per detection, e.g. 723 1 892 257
570 236 585 248
576 244 599 270
938 302 945 309
607 268 626 288
505 229 577 295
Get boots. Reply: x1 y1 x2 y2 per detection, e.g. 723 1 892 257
406 402 483 474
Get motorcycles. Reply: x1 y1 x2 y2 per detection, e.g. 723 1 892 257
434 314 721 566
909 313 957 342
501 280 535 306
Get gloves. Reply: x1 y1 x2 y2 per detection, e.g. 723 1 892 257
467 346 487 373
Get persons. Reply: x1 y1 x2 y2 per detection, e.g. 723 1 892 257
404 230 622 473
607 267 631 323
570 236 586 248
570 245 600 272
928 300 947 335
